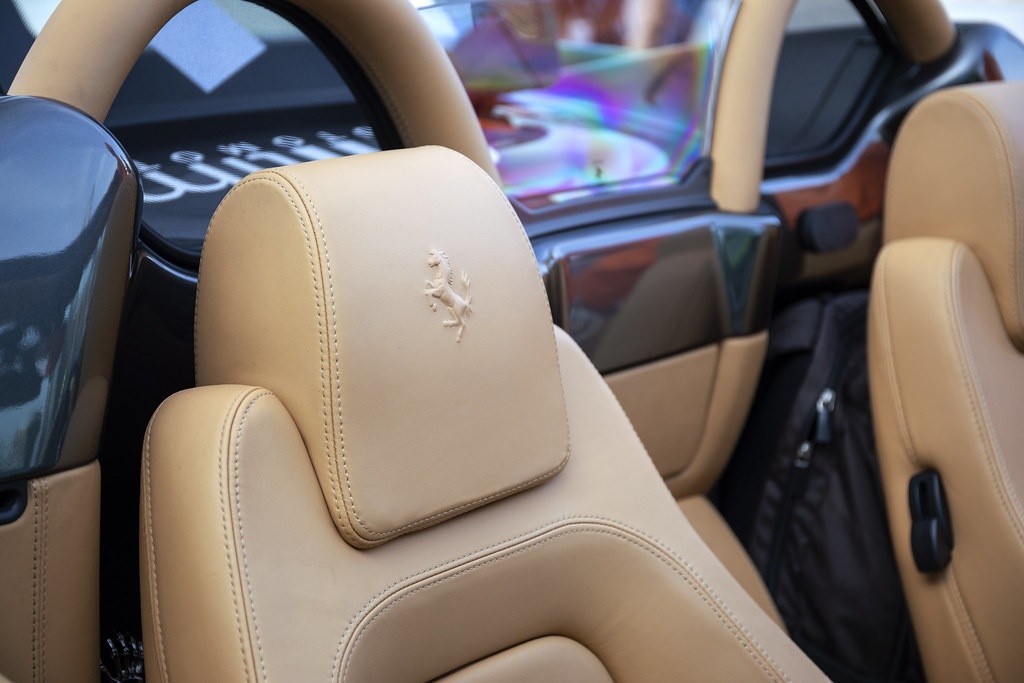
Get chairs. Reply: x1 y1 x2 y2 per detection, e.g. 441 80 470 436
0 91 1024 683
